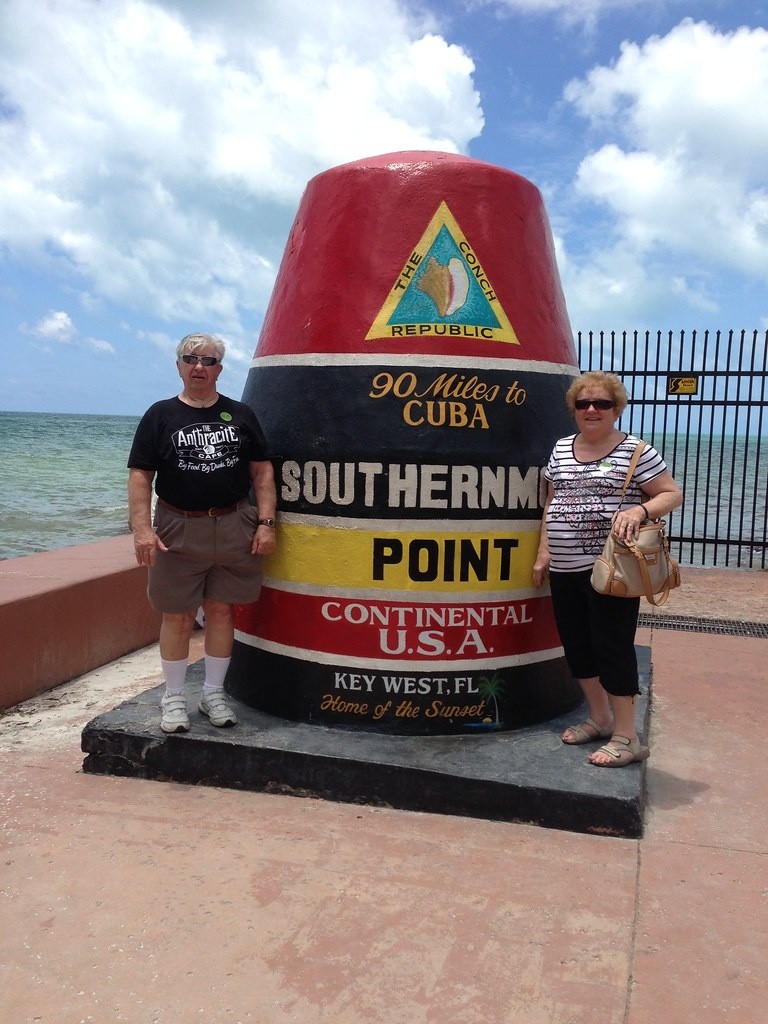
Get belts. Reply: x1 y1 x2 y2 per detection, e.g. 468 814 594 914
159 498 247 517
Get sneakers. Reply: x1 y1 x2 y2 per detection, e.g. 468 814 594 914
198 688 237 728
160 693 190 733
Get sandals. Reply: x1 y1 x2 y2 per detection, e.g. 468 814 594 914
561 717 614 745
588 735 650 768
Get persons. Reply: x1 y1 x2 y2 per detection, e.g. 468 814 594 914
128 333 276 733
532 371 682 767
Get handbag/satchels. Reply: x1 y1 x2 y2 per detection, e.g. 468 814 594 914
590 441 681 607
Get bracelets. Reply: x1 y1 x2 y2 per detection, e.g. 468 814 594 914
639 504 648 522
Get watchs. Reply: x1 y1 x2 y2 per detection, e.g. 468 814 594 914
258 518 276 527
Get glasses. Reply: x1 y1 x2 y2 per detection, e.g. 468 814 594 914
181 354 220 366
575 400 616 410
